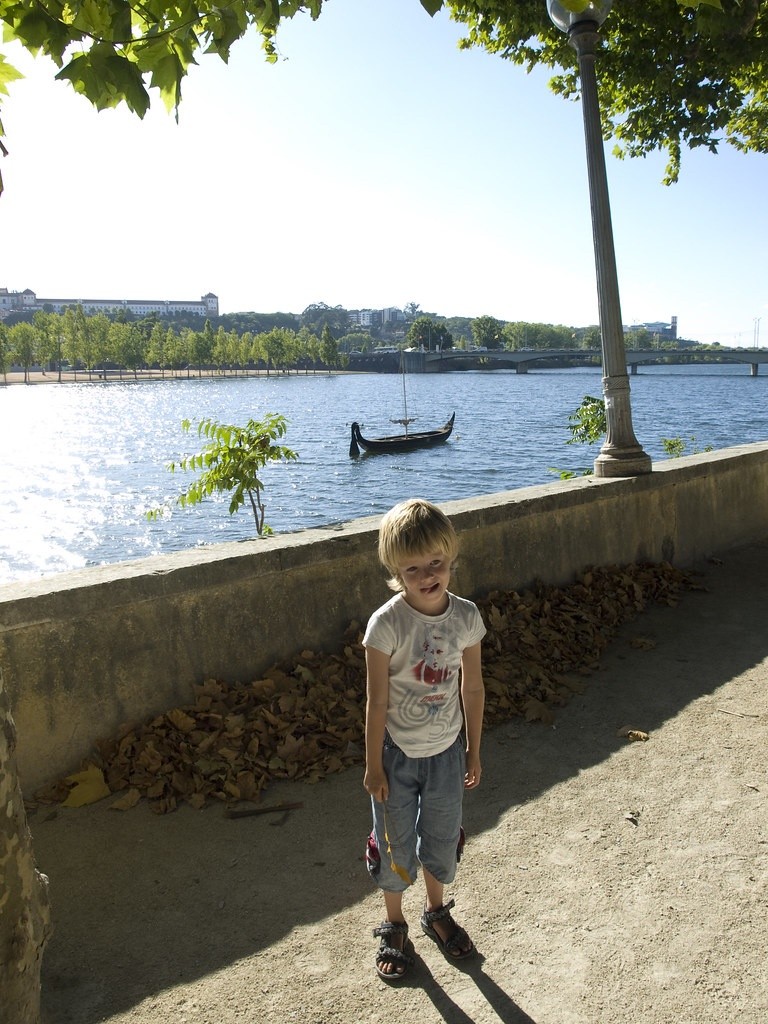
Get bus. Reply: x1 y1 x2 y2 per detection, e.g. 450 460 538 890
373 347 399 354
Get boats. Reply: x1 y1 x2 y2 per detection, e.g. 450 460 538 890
345 344 456 458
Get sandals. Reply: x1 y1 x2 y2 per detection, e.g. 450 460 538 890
373 917 415 979
421 900 475 960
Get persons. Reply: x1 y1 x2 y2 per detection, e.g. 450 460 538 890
356 493 485 981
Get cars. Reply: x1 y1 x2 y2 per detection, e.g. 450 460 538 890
521 347 535 351
452 347 465 352
349 351 361 354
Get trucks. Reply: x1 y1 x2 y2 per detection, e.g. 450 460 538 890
472 346 488 351
